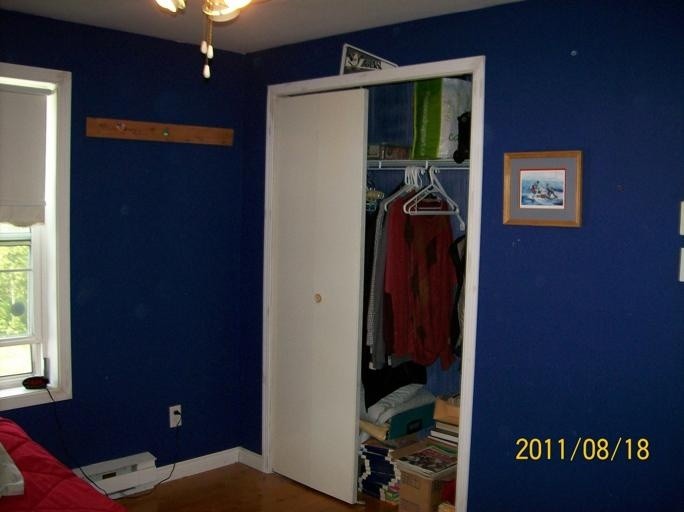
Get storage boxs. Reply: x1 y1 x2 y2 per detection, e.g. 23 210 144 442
394 438 457 508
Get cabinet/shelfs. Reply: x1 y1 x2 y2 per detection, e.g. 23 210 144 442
260 56 486 512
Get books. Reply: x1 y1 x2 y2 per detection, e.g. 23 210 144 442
358 421 461 510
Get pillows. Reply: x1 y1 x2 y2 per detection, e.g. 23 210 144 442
0 443 25 497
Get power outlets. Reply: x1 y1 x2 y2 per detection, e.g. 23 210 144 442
170 405 183 428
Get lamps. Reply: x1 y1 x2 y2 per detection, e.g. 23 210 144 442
155 0 250 79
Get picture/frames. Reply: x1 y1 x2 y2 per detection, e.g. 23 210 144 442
502 150 583 228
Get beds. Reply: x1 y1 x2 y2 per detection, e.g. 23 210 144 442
0 415 130 512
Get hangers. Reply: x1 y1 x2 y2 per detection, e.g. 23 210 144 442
366 164 460 219
